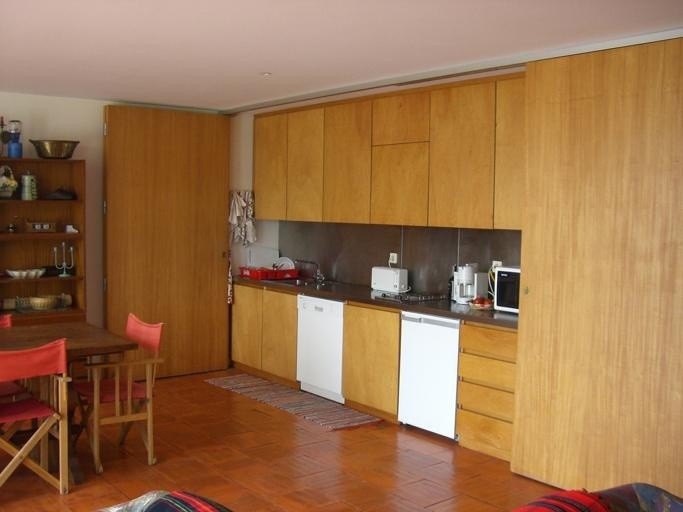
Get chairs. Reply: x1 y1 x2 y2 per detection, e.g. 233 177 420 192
0 312 164 495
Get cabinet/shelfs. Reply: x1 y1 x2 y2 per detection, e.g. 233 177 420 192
429 74 498 230
231 284 299 390
253 95 326 224
342 301 401 424
323 94 374 224
493 71 525 230
0 158 87 326
455 321 518 462
371 85 429 228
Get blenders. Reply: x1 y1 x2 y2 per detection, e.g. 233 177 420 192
448 263 490 306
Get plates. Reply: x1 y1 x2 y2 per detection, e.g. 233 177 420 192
6 268 46 279
270 256 296 270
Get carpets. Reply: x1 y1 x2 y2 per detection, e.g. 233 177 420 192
204 373 383 432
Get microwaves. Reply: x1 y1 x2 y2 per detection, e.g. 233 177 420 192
493 265 520 315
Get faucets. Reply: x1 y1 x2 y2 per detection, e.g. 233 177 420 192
294 258 325 285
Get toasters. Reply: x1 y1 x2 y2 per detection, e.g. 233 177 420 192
370 265 409 294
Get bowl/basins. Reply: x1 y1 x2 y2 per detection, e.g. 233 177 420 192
28 137 80 160
30 294 59 310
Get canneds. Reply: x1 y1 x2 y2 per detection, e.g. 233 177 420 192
21 175 38 201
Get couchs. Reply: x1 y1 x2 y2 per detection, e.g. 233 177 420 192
510 483 682 511
96 489 231 511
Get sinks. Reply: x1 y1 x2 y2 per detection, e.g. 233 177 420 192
277 280 349 288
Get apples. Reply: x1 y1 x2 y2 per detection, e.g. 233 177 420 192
474 296 491 306
